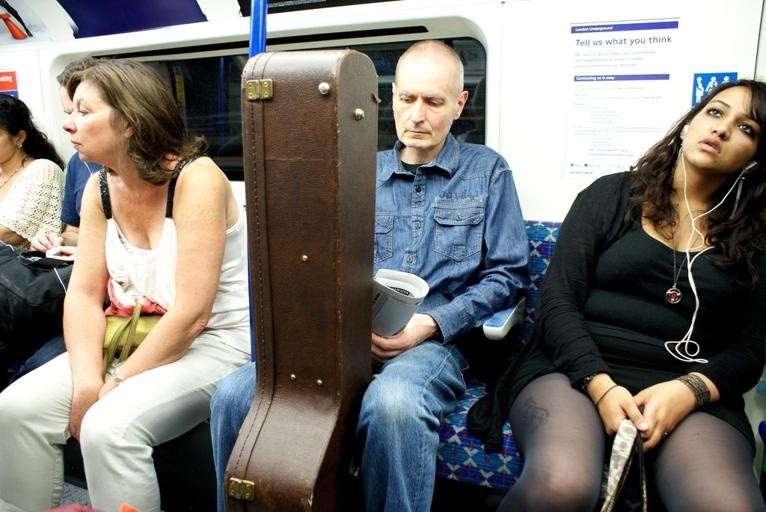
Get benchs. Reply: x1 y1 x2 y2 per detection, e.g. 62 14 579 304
439 210 561 491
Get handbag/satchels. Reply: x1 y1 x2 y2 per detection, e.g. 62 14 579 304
103 315 164 356
0 239 74 374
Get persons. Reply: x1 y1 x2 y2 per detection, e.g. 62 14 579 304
208 40 530 511
465 80 766 511
1 57 101 392
2 60 252 512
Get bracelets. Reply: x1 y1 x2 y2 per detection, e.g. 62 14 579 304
677 372 711 411
111 368 125 387
595 384 620 411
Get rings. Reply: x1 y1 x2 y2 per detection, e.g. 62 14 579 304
661 431 669 441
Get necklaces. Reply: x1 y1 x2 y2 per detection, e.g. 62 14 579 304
664 215 706 304
